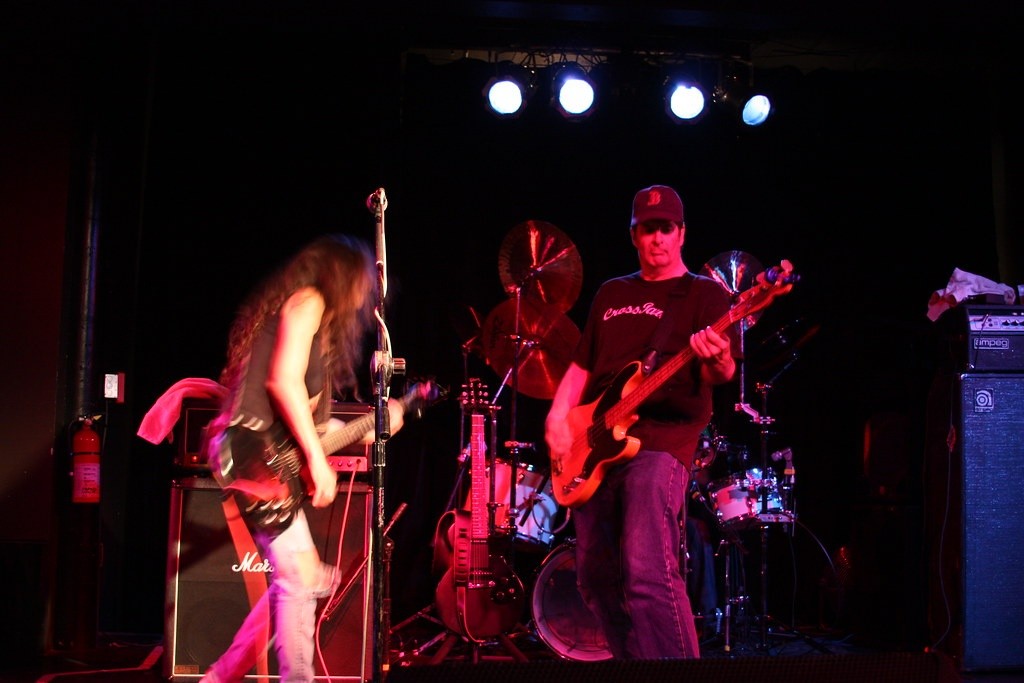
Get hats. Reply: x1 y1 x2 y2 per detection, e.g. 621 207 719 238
631 185 684 227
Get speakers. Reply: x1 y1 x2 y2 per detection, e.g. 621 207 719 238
162 473 375 683
953 373 1024 676
383 648 960 683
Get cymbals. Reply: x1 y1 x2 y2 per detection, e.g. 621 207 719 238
481 296 582 400
499 219 585 313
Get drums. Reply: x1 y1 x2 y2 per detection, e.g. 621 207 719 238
533 539 618 661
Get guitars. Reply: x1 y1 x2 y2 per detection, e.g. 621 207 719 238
437 377 527 641
208 379 441 536
543 254 795 504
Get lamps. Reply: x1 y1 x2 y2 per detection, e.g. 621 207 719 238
716 73 774 128
656 64 712 126
480 59 531 121
545 61 601 119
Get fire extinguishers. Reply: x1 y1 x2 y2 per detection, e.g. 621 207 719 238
70 414 101 504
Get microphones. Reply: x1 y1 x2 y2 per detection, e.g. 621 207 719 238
366 187 389 214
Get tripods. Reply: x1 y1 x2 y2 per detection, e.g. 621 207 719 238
693 318 838 657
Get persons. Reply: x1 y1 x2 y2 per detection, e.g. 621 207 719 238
200 233 406 683
544 185 743 664
